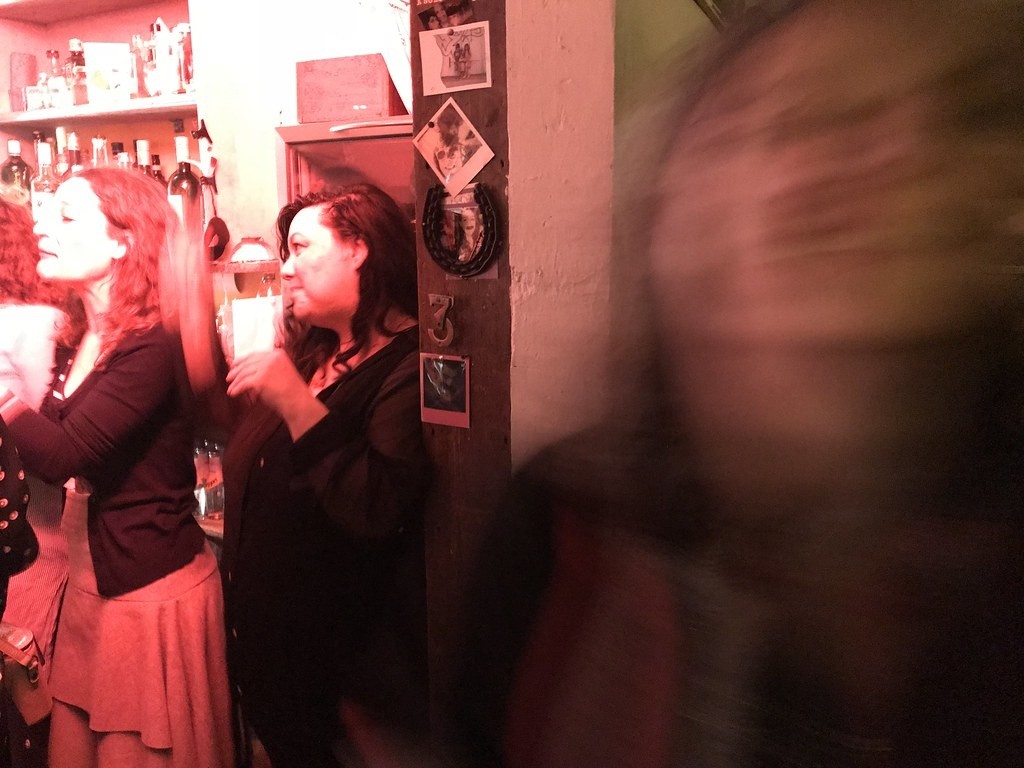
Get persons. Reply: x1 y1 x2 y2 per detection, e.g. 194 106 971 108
0 168 234 768
451 208 480 264
422 2 464 30
433 106 481 180
0 198 66 623
222 183 423 768
461 0 1024 768
453 43 472 80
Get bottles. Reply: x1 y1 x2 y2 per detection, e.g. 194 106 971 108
193 437 226 523
256 272 280 300
0 124 203 238
129 18 195 98
19 38 86 113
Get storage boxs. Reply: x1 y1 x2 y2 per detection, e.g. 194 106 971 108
293 52 408 124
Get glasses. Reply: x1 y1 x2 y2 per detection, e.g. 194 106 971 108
436 150 458 160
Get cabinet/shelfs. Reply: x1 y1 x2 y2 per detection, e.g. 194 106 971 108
0 1 208 229
275 114 419 343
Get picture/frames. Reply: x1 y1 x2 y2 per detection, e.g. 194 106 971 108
420 181 501 279
